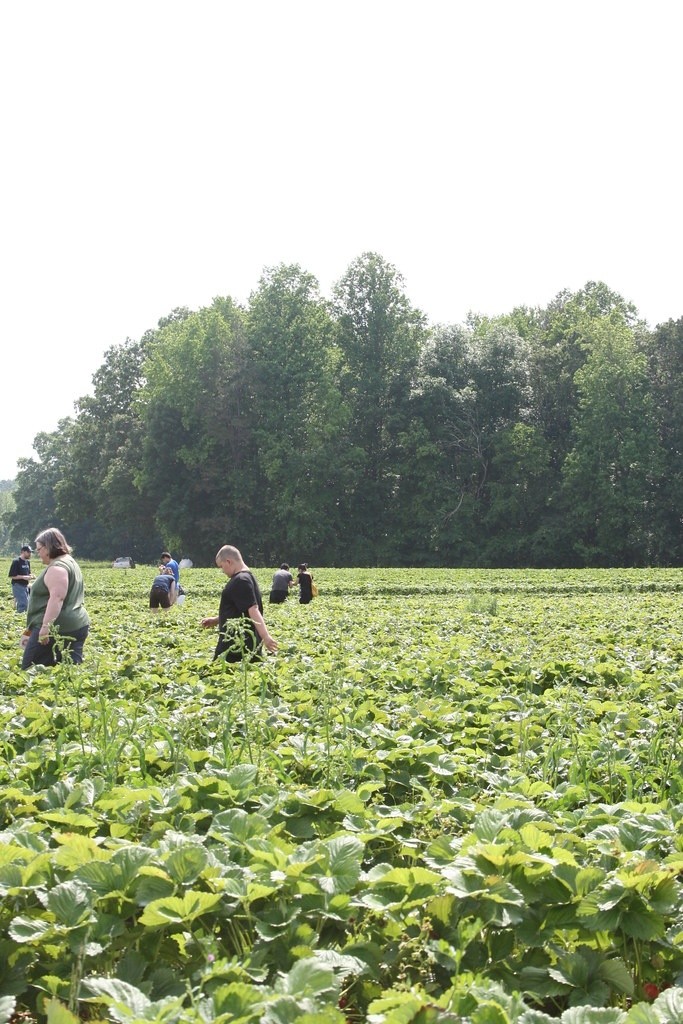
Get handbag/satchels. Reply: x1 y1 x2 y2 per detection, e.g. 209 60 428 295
311 580 318 596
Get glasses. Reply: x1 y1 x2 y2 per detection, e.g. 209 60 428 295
35 545 46 553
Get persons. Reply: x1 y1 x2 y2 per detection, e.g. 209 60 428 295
8 546 36 611
293 565 313 604
201 545 278 663
22 528 89 671
150 552 185 609
270 563 294 603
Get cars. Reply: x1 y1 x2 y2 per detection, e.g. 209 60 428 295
113 556 135 570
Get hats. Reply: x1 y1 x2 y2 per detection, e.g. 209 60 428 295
21 546 33 552
